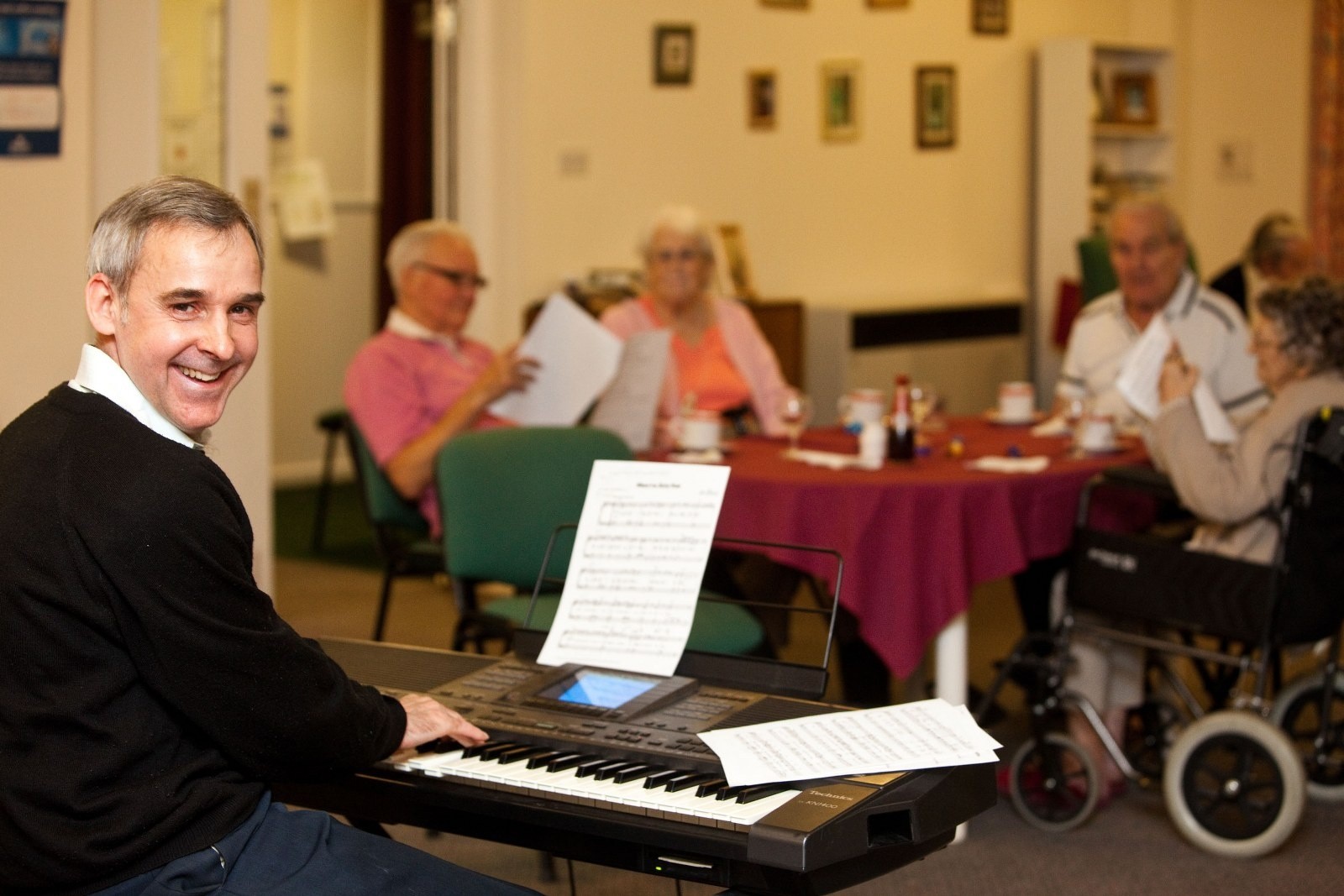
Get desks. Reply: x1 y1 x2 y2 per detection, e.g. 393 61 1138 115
642 417 1152 845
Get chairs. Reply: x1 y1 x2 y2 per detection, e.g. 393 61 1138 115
303 407 770 881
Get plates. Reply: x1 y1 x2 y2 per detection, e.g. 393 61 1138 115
986 411 1047 425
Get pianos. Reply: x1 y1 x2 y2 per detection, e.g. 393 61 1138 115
270 632 998 896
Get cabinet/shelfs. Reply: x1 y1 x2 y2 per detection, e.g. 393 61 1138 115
1031 36 1177 410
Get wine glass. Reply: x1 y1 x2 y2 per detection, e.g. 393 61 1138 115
777 395 817 464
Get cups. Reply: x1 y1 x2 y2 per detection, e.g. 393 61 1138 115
998 381 1036 418
837 387 885 427
667 410 720 451
1086 409 1115 447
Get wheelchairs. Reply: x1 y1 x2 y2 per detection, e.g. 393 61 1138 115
1003 401 1344 860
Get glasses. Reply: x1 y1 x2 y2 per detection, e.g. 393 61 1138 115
409 260 487 297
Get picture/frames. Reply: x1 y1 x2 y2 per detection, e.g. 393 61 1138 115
745 66 779 132
912 63 960 149
818 60 861 145
650 20 698 88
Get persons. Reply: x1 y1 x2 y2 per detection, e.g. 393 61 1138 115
595 213 893 708
341 218 544 544
0 177 545 896
1012 193 1343 805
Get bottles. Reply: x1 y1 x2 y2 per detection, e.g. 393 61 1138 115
891 375 914 461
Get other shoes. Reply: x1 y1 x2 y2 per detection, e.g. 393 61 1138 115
994 634 1054 680
1065 765 1126 806
